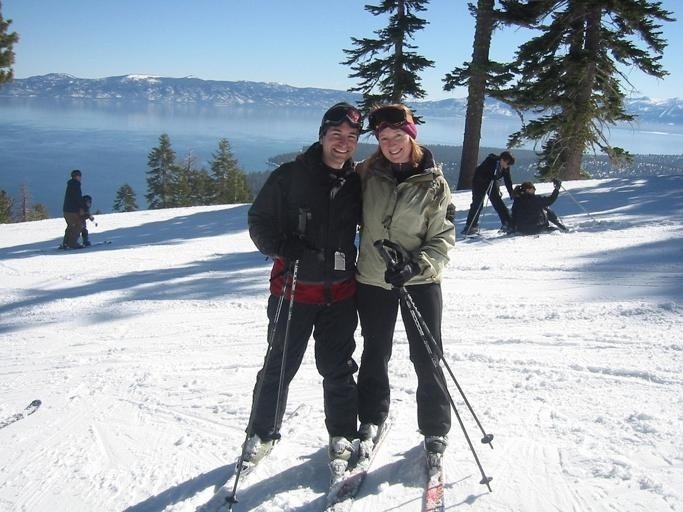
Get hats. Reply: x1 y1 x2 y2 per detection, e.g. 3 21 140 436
319 102 362 137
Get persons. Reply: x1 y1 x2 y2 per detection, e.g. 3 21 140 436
80 195 94 246
463 150 514 234
351 102 454 452
243 101 361 461
63 170 84 248
508 178 568 234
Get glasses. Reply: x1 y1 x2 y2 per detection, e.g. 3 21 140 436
369 106 412 130
322 107 363 125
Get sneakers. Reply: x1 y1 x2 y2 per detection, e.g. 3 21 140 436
242 434 278 464
358 420 386 442
329 435 352 461
63 241 91 248
425 436 448 453
464 225 478 232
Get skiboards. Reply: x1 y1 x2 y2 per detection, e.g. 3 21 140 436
0 399 41 429
206 401 447 511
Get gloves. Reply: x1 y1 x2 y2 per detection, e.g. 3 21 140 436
446 203 455 223
385 262 421 287
555 180 561 189
273 238 307 258
